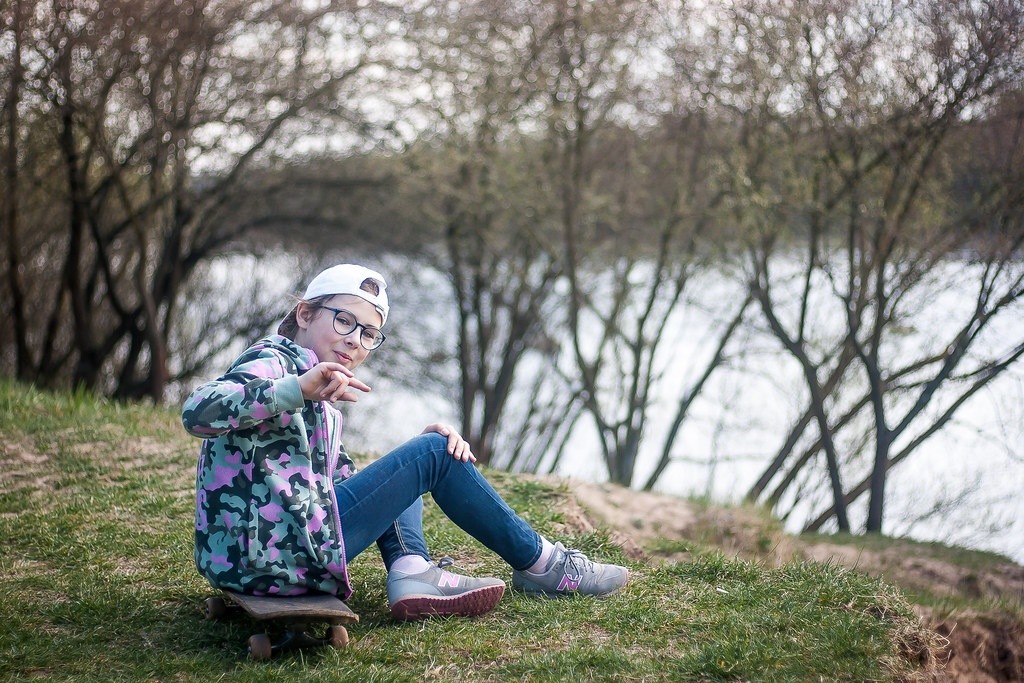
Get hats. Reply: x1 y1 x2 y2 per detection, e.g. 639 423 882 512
276 264 390 341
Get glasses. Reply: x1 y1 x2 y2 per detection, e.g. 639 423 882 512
318 302 387 351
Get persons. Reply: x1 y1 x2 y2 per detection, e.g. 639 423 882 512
182 264 629 620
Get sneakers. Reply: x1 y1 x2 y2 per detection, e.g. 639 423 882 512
386 556 507 625
513 540 630 599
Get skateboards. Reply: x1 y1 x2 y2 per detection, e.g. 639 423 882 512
205 578 360 660
